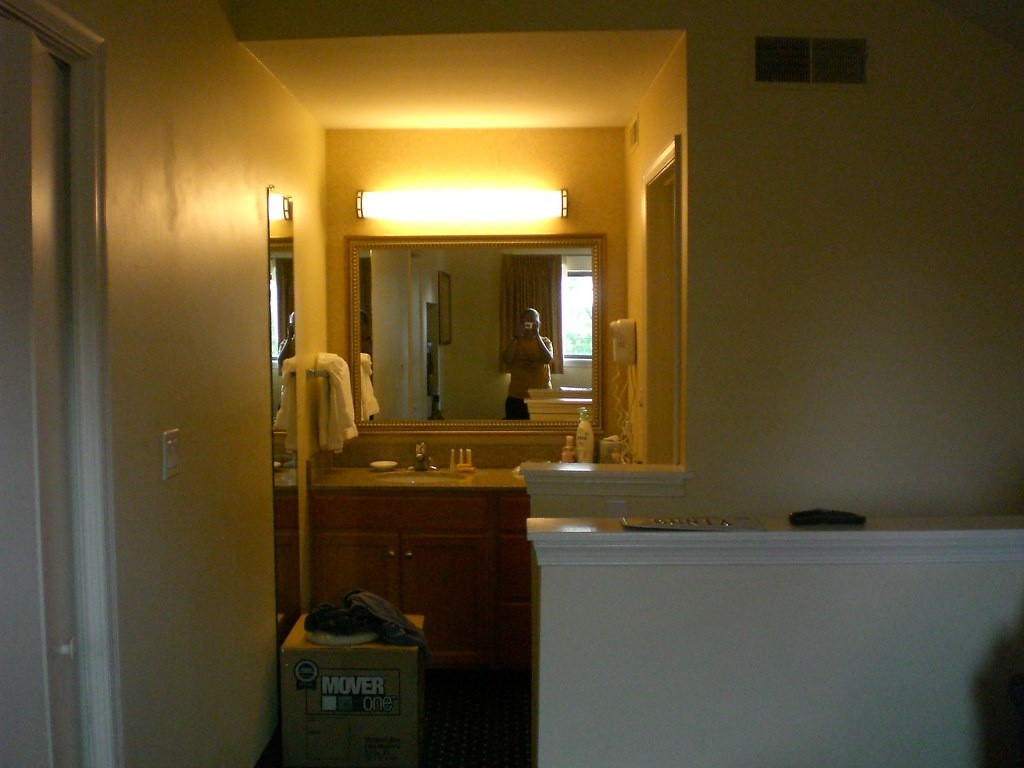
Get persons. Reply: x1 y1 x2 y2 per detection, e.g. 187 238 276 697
503 308 553 419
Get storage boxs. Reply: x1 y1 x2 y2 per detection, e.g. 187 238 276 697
280 614 425 768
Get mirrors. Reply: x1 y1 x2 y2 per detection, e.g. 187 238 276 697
343 232 608 436
358 249 374 421
268 187 301 726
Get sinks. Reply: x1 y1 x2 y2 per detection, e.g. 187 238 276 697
374 470 473 485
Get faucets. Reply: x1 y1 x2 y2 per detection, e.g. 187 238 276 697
407 441 438 471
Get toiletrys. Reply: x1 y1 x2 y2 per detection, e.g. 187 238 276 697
561 407 596 463
447 448 476 473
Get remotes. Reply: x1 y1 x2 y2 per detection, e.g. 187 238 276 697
789 509 865 526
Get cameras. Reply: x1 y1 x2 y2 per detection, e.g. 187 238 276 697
525 321 534 328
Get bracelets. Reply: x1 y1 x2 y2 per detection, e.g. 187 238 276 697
513 336 519 344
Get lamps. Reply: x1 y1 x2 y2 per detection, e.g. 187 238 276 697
356 189 568 219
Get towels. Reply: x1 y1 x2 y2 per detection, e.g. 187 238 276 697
359 352 381 422
273 356 296 455
316 351 360 454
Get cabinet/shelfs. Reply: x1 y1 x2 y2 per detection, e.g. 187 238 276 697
496 491 531 670
308 491 496 670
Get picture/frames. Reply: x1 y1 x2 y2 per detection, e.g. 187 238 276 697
438 271 452 345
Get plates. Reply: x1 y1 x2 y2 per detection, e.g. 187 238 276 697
370 461 398 470
